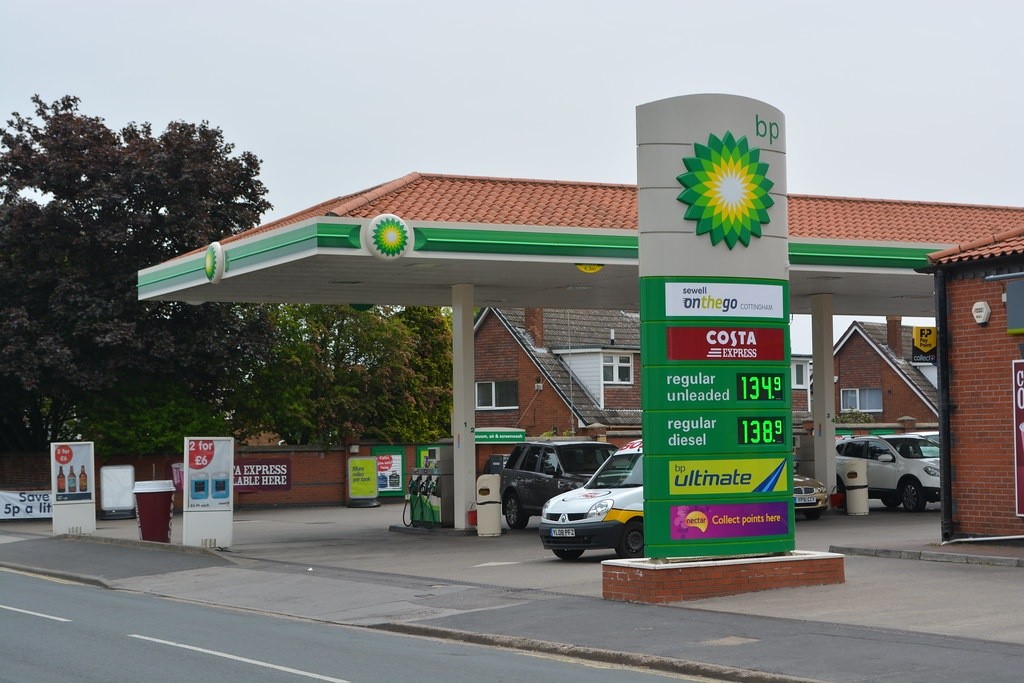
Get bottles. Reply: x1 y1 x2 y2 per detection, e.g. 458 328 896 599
57 466 65 492
79 465 87 491
68 466 76 492
377 472 387 487
389 470 400 487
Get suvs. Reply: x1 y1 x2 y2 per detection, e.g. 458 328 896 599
834 432 941 512
498 441 621 530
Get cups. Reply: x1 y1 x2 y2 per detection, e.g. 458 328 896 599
134 480 175 543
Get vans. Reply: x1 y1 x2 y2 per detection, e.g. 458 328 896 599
538 438 644 560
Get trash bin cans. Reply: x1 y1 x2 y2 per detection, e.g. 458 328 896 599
475 474 502 537
843 459 870 516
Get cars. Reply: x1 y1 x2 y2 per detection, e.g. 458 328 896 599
793 473 829 520
